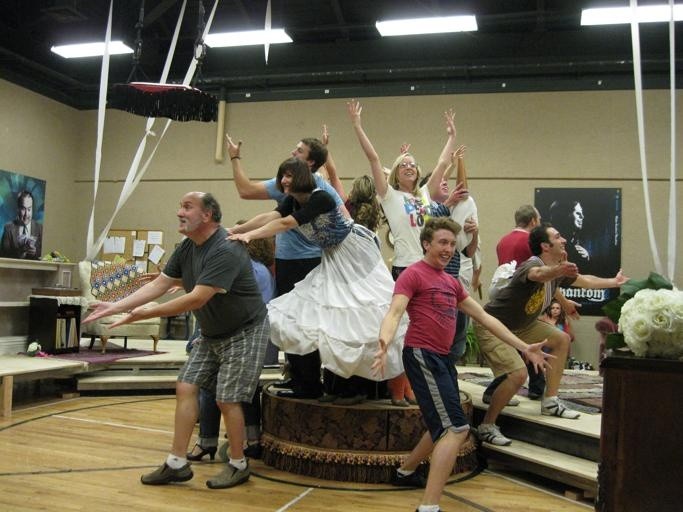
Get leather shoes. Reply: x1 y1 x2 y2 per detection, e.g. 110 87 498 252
392 398 417 406
274 378 366 405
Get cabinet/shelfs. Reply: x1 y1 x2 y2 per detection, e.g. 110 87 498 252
597 350 679 511
29 296 81 353
1 257 78 355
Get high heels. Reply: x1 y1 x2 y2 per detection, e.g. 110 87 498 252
186 443 218 460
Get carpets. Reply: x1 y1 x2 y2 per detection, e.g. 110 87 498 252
458 368 604 415
26 340 167 366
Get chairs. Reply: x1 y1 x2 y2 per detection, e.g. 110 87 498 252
80 260 160 354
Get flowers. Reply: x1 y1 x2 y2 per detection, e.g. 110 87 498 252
602 273 682 360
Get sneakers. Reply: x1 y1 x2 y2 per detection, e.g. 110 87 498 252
141 462 193 483
528 392 580 418
477 423 512 446
206 458 249 488
392 469 429 487
483 392 520 405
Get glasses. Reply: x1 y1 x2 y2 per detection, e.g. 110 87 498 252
399 162 416 168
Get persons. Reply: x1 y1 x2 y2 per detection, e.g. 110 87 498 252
371 214 560 512
78 189 267 489
1 187 41 264
